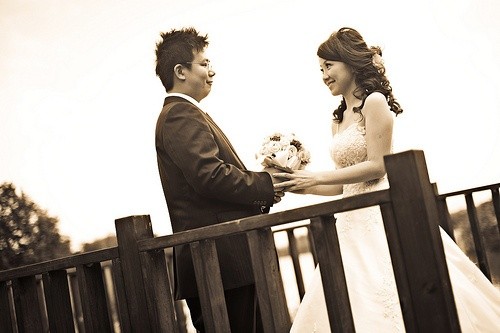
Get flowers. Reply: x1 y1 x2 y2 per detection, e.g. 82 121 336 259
256 132 310 174
371 54 384 69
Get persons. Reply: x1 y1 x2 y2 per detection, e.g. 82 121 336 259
273 27 500 333
155 28 290 333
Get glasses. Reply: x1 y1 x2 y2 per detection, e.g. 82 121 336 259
185 60 212 70
